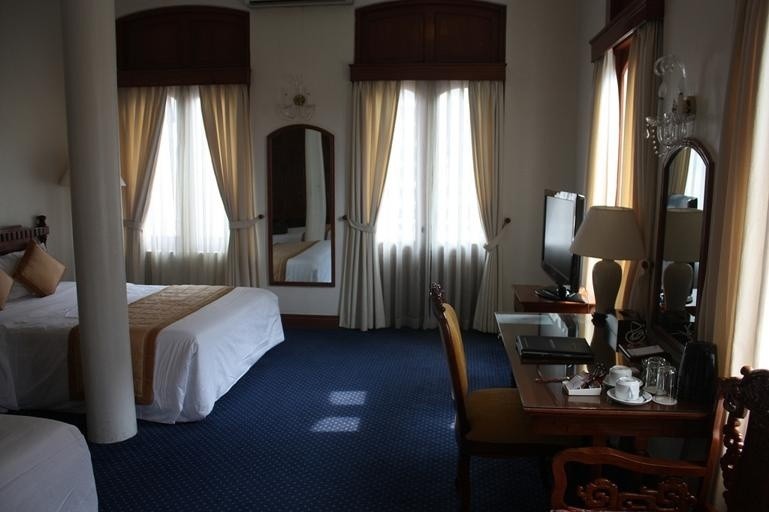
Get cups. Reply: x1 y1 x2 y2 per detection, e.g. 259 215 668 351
656 366 677 403
645 357 665 394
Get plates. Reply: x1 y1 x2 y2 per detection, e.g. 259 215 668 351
652 396 677 406
562 381 602 396
603 377 643 388
606 387 652 406
643 386 667 395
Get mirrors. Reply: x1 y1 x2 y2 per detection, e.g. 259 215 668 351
645 137 715 363
264 124 334 285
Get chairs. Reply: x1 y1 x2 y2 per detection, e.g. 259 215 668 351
431 280 594 512
552 366 767 512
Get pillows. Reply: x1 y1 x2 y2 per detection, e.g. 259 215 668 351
17 242 68 294
0 272 12 310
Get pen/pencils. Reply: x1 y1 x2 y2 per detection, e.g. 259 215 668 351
533 376 570 384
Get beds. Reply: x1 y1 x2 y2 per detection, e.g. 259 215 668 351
0 215 286 424
0 412 100 511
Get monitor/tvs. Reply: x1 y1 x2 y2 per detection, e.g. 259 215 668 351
534 189 586 303
661 194 697 304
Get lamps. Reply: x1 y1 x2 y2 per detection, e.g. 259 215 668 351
570 206 644 316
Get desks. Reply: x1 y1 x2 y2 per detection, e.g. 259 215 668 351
495 312 708 512
511 284 590 316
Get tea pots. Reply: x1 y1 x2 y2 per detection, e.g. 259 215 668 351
609 365 632 385
615 376 643 401
